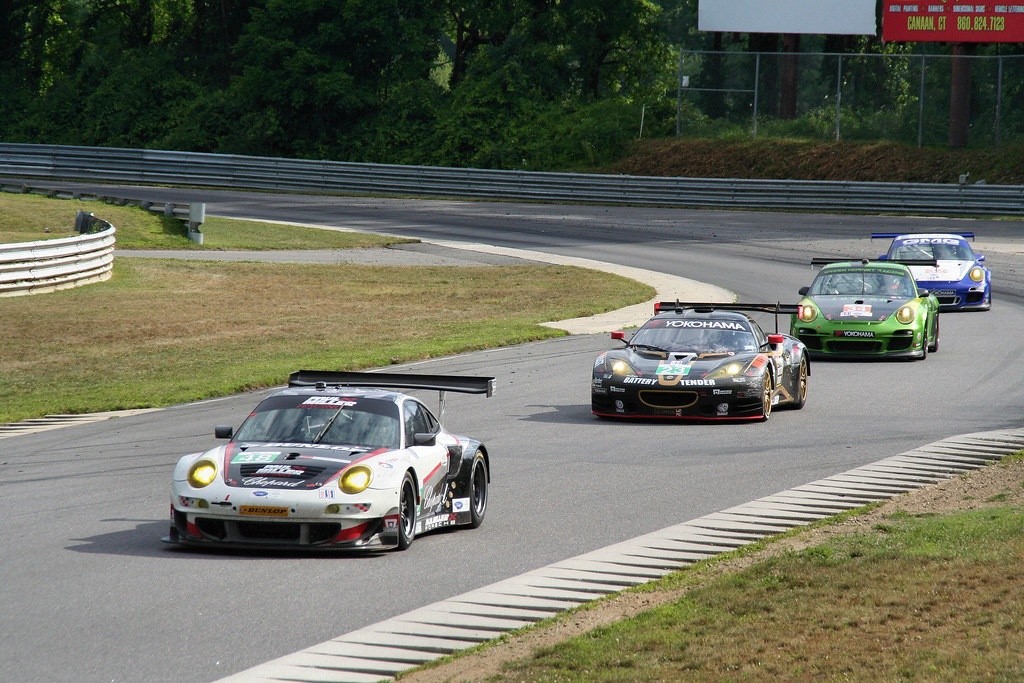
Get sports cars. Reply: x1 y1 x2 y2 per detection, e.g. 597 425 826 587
871 231 993 313
591 301 811 422
788 257 940 360
164 369 496 552
